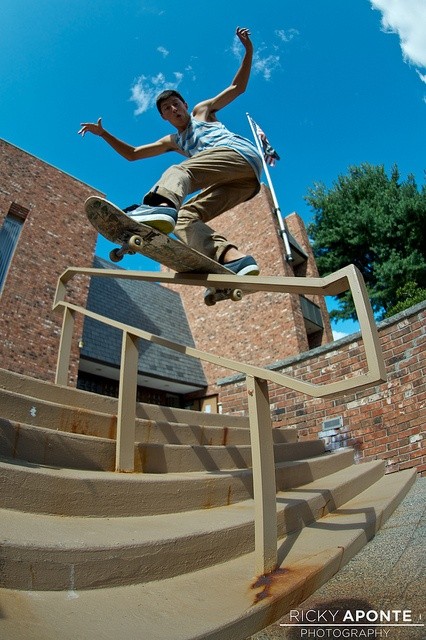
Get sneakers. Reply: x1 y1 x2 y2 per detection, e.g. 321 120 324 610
202 255 259 302
124 205 177 233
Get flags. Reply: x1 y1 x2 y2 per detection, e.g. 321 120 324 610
249 118 280 167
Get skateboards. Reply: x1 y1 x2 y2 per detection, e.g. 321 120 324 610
84 194 259 307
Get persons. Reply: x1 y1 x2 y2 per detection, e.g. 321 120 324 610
77 26 263 275
